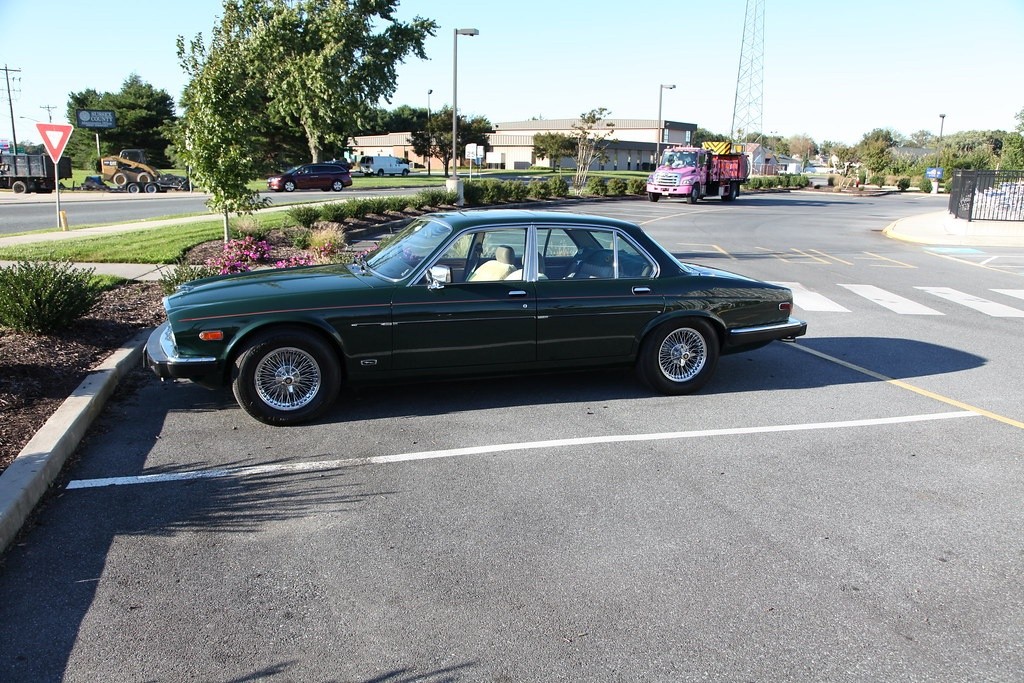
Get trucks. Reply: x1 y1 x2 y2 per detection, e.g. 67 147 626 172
0 148 72 194
645 145 748 204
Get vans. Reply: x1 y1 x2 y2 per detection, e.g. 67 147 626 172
360 156 411 178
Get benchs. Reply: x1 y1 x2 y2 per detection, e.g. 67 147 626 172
561 249 645 280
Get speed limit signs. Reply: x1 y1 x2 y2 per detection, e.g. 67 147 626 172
465 142 476 160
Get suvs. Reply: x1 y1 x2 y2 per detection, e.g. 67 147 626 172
267 162 352 192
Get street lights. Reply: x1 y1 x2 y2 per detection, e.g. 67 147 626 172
930 114 946 194
446 28 480 207
655 84 676 170
428 89 433 175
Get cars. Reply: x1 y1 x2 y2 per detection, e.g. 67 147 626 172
144 208 808 426
329 161 353 172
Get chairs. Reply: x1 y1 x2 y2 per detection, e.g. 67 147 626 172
468 246 517 281
506 252 549 280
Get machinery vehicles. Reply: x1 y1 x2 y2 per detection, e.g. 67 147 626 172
100 149 186 194
